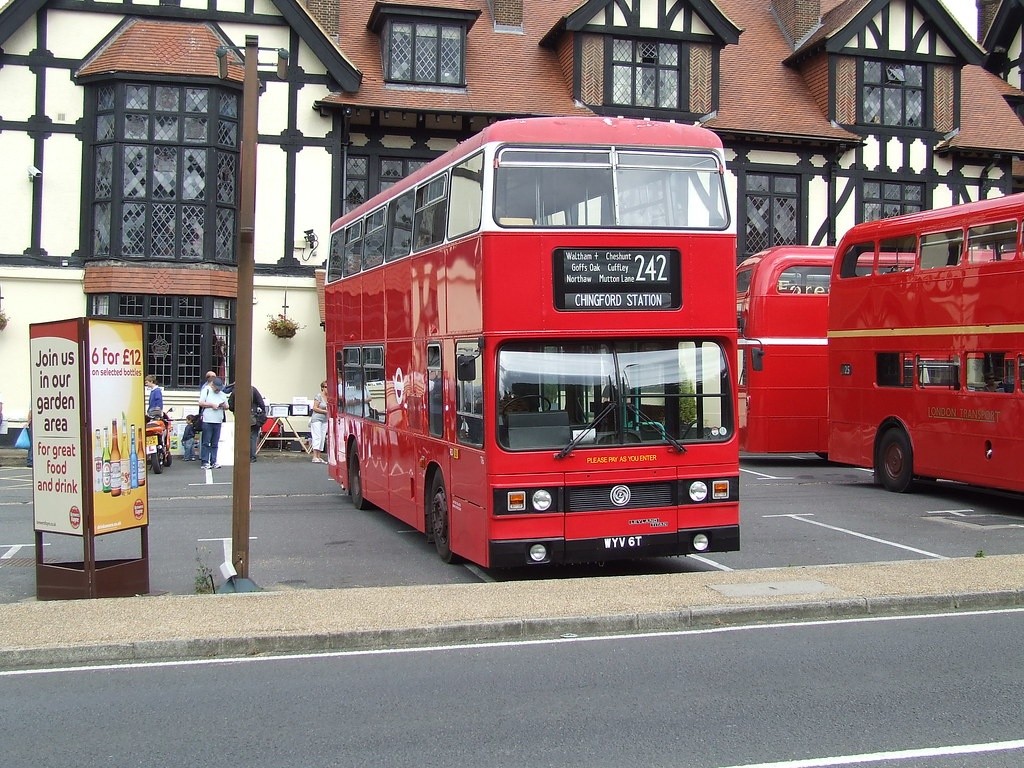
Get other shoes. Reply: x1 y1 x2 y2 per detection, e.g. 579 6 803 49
182 457 189 461
192 457 196 460
312 458 321 462
250 456 256 462
319 458 325 462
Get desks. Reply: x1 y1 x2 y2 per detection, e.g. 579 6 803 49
255 416 310 454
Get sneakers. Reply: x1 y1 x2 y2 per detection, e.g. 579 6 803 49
201 463 212 469
211 463 221 468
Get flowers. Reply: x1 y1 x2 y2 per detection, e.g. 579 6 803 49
265 313 307 338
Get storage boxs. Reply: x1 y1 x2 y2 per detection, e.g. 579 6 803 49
269 403 290 417
264 405 269 415
291 404 310 415
290 441 302 453
262 417 280 435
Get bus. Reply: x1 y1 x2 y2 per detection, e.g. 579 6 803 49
324 117 765 572
736 192 1024 494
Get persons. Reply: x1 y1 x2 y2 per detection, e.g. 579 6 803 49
501 378 540 413
181 414 197 461
145 374 164 422
197 370 232 470
228 386 266 462
26 408 33 467
338 373 372 418
310 380 329 464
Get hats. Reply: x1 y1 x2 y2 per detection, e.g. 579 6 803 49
213 378 223 389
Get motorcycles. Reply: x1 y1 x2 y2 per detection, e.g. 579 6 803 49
146 407 173 474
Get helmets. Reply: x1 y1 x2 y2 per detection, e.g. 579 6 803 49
206 371 216 378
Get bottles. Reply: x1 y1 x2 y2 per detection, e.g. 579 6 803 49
130 424 138 489
103 427 110 492
137 428 146 486
110 421 121 496
120 419 130 496
94 429 103 492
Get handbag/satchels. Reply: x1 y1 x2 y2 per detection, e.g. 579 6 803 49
195 414 202 431
256 414 268 427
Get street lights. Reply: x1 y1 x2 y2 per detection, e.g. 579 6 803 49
216 32 290 594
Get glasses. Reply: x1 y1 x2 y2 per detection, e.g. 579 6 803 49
324 386 327 388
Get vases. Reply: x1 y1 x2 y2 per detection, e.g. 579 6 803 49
278 329 295 337
